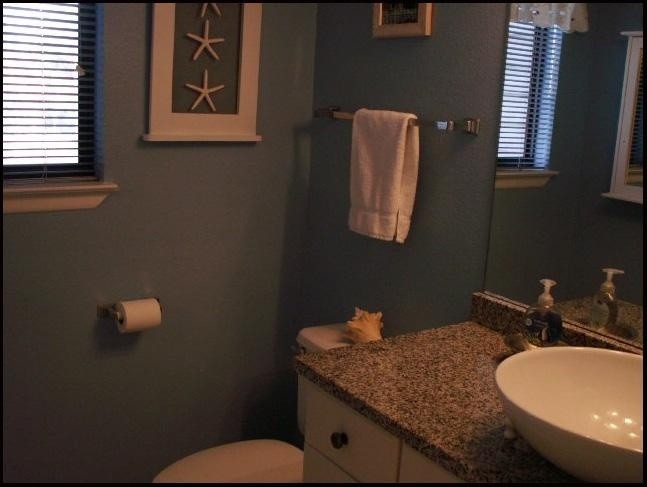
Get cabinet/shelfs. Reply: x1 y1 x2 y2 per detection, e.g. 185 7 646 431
300 385 463 484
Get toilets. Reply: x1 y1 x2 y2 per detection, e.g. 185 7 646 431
152 323 353 483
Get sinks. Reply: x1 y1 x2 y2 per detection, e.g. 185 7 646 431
495 346 643 483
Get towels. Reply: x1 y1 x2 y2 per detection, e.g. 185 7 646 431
348 106 420 244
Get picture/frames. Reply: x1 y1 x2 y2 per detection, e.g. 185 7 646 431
371 3 432 40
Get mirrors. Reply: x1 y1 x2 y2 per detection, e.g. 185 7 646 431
483 5 644 349
